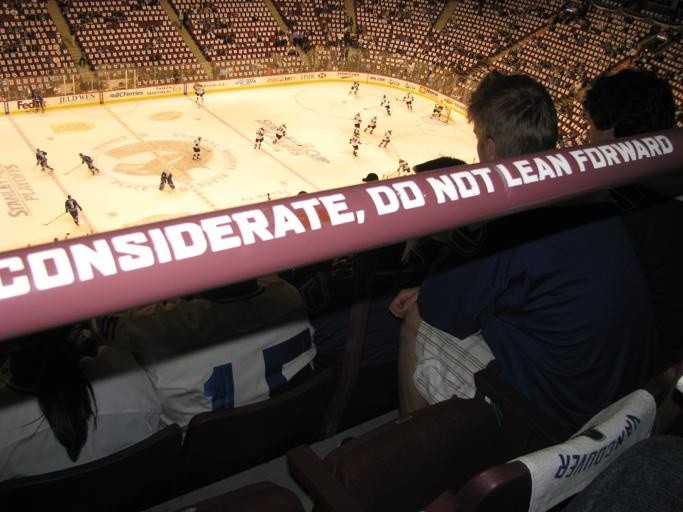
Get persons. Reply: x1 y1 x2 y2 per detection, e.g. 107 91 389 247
79 153 99 175
65 194 82 226
399 159 490 271
95 275 309 363
159 170 175 191
398 157 410 173
298 246 440 323
1 0 683 160
362 173 379 182
389 68 656 442
580 68 683 388
2 322 141 462
54 237 58 242
36 148 54 172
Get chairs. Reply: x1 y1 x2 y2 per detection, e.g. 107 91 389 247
0 291 683 512
